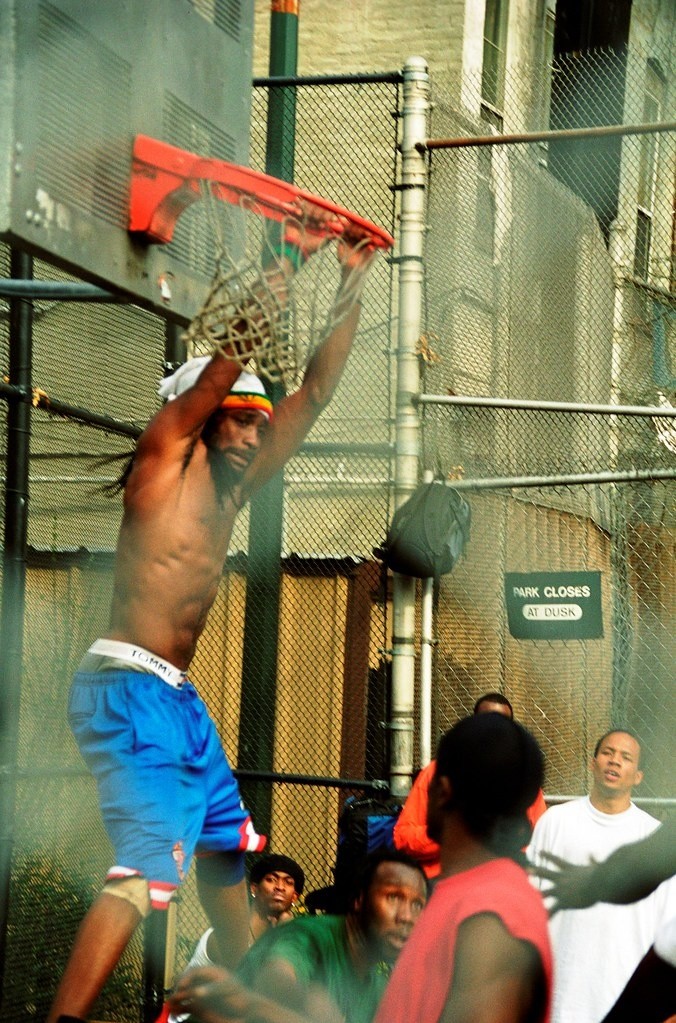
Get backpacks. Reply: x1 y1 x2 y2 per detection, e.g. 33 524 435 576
372 476 471 616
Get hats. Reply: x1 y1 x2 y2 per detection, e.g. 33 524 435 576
156 356 275 427
250 854 305 895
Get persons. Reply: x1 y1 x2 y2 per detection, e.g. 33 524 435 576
522 730 675 1021
390 692 549 888
184 853 433 1021
43 199 372 1022
167 853 304 1023
524 807 676 1023
171 711 556 1023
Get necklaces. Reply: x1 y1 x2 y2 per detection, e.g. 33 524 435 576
250 927 258 945
